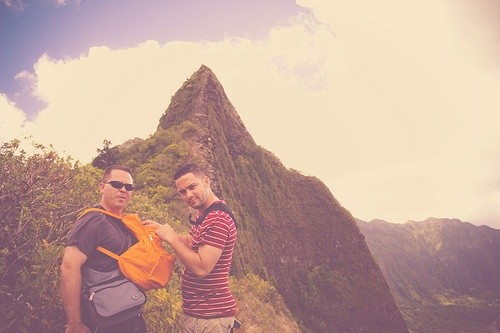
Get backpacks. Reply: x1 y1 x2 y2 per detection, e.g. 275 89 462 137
78 209 177 290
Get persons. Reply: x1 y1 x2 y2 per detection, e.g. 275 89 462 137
58 165 147 333
140 163 236 333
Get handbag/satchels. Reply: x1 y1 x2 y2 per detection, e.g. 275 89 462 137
82 276 146 326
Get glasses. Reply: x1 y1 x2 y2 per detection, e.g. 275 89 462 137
105 181 134 192
